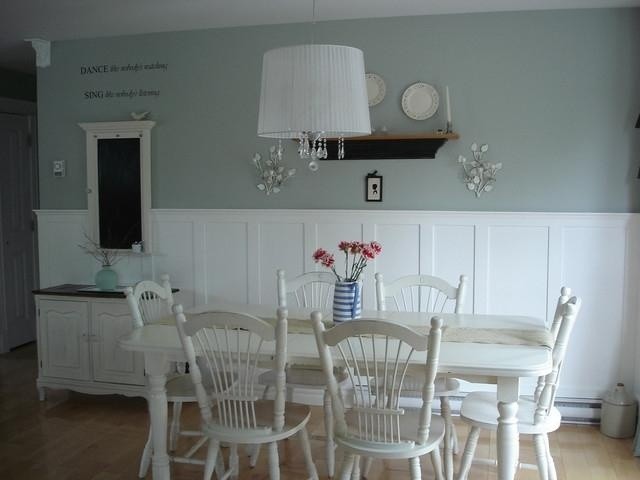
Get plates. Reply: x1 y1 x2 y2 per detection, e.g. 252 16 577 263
401 82 440 122
365 73 387 107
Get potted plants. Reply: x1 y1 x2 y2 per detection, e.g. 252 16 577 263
77 234 139 290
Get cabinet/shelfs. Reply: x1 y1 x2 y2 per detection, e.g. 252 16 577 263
36 296 168 401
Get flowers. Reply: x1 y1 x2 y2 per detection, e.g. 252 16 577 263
312 240 382 282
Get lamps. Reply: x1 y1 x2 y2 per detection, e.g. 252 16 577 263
258 0 371 170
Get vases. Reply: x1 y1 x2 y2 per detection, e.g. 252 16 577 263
333 283 361 324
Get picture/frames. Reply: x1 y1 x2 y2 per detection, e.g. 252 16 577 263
365 176 383 202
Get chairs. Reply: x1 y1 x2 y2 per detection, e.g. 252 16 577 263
249 270 363 474
121 273 227 479
309 310 446 479
458 286 581 480
172 305 318 480
369 273 468 454
